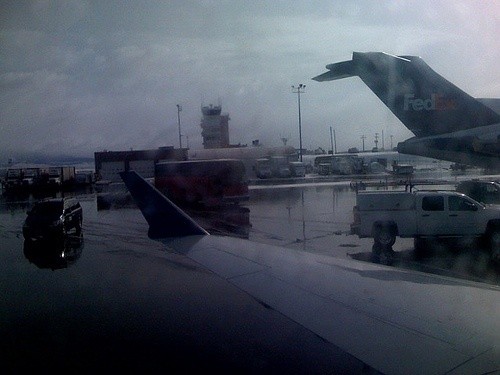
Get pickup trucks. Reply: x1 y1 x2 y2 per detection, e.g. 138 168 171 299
407 179 500 241
354 190 499 248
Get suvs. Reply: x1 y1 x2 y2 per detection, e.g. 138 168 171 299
21 197 85 240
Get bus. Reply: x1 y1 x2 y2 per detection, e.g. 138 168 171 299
154 158 248 199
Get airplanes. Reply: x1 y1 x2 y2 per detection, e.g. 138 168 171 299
312 51 500 170
116 171 500 374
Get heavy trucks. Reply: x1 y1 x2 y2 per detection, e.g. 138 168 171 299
6 167 22 186
22 168 50 187
49 166 76 185
313 154 388 174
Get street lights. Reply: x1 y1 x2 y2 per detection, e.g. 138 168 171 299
175 103 187 147
291 82 307 164
